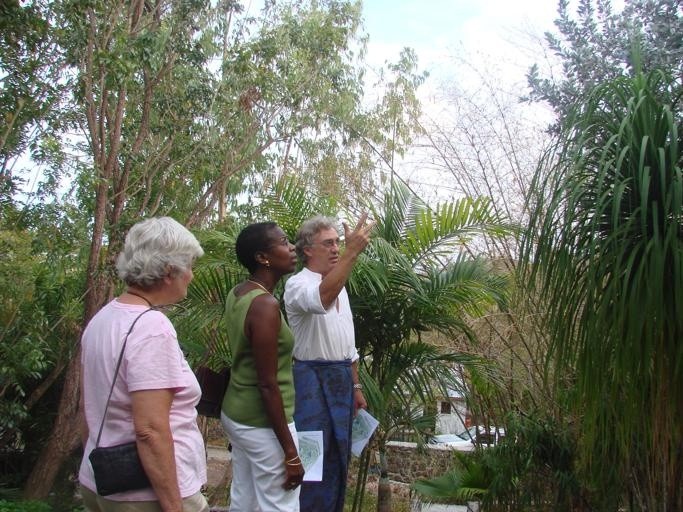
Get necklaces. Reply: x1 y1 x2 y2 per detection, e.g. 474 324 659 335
127 291 152 307
247 279 273 296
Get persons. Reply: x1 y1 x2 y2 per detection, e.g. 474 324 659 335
283 212 378 512
220 222 305 512
78 216 210 512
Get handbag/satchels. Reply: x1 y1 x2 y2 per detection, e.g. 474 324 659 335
194 363 231 418
88 440 153 496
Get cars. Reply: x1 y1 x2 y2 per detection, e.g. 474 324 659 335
428 425 506 447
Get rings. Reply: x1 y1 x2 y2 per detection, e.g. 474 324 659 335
291 482 296 486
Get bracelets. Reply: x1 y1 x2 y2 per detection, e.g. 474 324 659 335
354 384 362 390
285 456 302 466
